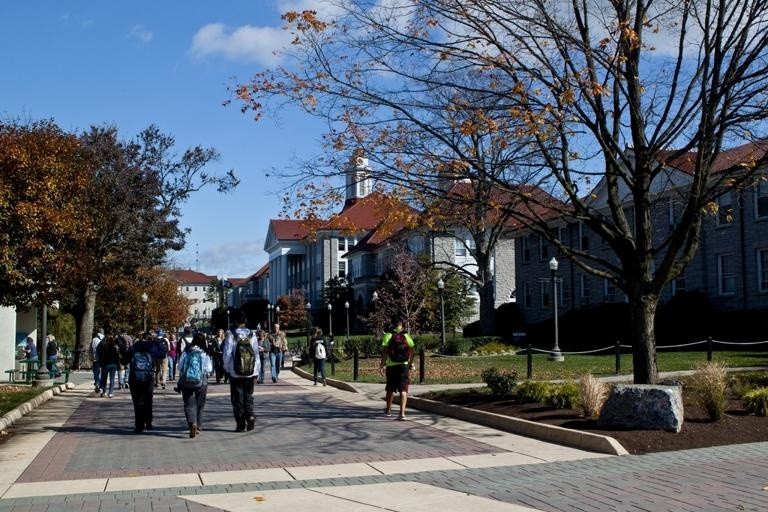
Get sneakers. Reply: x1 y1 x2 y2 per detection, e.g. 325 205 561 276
190 422 200 438
234 412 256 432
109 394 114 398
119 382 130 390
101 392 107 397
323 379 326 386
134 422 153 433
95 384 102 393
155 381 167 389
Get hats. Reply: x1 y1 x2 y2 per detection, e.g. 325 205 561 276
98 328 105 334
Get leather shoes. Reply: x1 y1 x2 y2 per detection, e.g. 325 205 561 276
174 387 181 393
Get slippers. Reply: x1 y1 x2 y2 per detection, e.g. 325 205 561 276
381 411 407 420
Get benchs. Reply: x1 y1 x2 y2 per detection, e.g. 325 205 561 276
4 357 72 384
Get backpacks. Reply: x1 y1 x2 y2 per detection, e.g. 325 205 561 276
130 351 153 382
155 337 169 352
315 343 328 359
230 329 255 377
182 336 191 346
180 350 204 388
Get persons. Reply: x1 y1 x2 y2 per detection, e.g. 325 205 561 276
89 309 288 440
21 334 61 379
380 319 416 420
309 327 328 386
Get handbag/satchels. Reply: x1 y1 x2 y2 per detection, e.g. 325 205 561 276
388 329 410 362
167 342 178 358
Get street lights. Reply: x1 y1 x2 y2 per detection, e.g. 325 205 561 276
140 291 150 334
28 242 56 387
436 276 447 349
265 303 281 333
226 309 231 329
304 301 312 346
344 300 350 339
546 256 566 362
370 290 380 302
326 302 334 345
